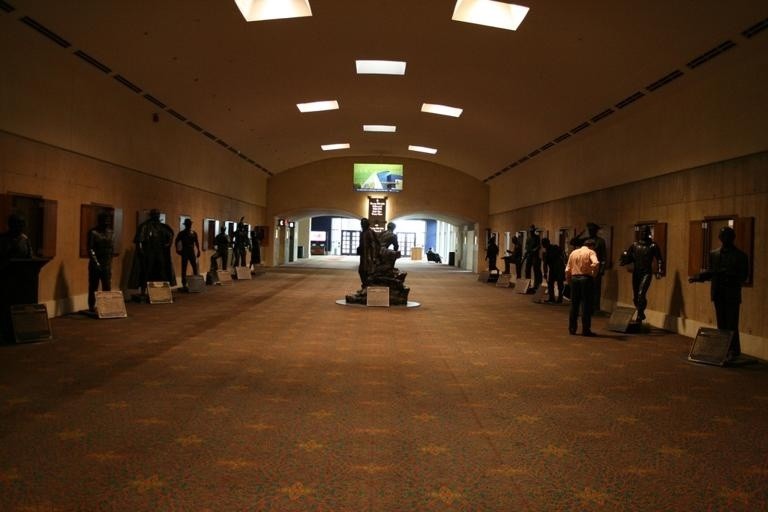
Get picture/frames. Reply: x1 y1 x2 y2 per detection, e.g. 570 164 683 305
94 266 252 319
367 286 390 307
496 274 548 303
605 306 635 332
688 328 735 367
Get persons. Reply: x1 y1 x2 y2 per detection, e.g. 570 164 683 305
210 225 231 271
428 247 442 263
250 230 262 267
502 236 523 278
175 218 202 294
358 217 379 288
619 224 664 323
230 221 248 268
0 212 35 258
86 210 121 311
566 239 601 338
377 221 401 260
568 222 607 313
687 225 750 357
133 207 177 295
484 236 499 270
523 225 543 288
541 238 565 302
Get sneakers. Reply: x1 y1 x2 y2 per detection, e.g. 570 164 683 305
568 329 596 337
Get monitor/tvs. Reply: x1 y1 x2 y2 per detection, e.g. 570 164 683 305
352 162 404 193
310 230 327 243
